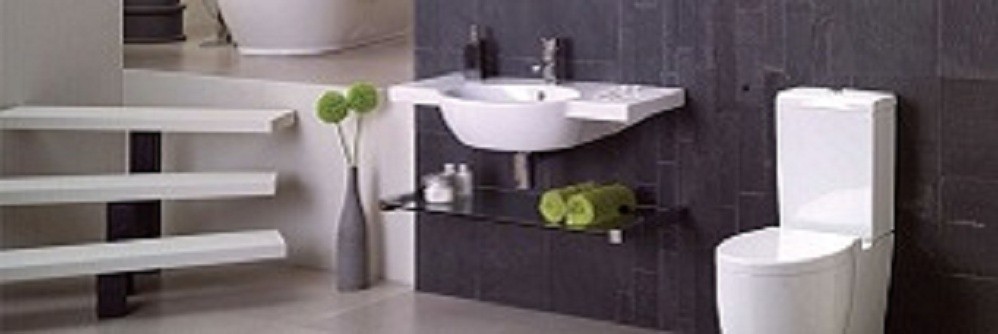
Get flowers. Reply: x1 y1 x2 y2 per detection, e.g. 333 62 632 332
317 80 377 166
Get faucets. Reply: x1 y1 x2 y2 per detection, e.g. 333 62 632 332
527 34 566 86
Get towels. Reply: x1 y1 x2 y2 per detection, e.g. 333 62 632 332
537 183 595 224
566 182 637 225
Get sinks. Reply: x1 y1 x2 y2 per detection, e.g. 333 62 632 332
441 76 582 154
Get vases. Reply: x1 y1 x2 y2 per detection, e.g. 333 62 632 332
335 166 370 292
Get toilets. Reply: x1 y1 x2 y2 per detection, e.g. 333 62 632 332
713 86 899 333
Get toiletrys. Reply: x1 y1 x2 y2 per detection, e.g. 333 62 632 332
423 162 474 204
463 23 484 81
